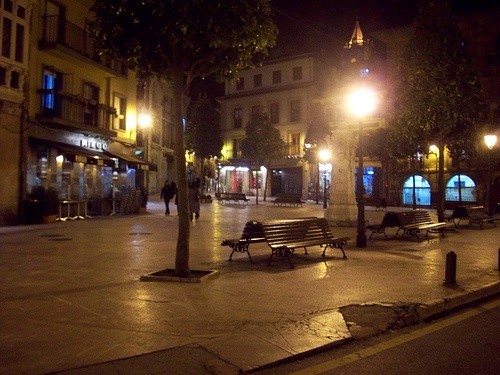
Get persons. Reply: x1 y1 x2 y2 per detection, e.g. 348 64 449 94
160 176 201 222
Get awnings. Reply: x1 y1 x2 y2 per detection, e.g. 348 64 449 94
28 136 116 168
101 148 159 172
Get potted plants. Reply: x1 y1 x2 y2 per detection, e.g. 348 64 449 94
26 184 62 223
135 186 148 215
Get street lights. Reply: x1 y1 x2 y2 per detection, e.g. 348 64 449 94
305 135 330 210
142 115 153 195
350 96 370 248
483 132 498 222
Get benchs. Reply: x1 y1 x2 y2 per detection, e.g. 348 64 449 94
441 203 497 230
271 192 306 208
221 216 317 263
214 192 250 206
397 209 447 241
256 218 351 269
366 212 420 241
